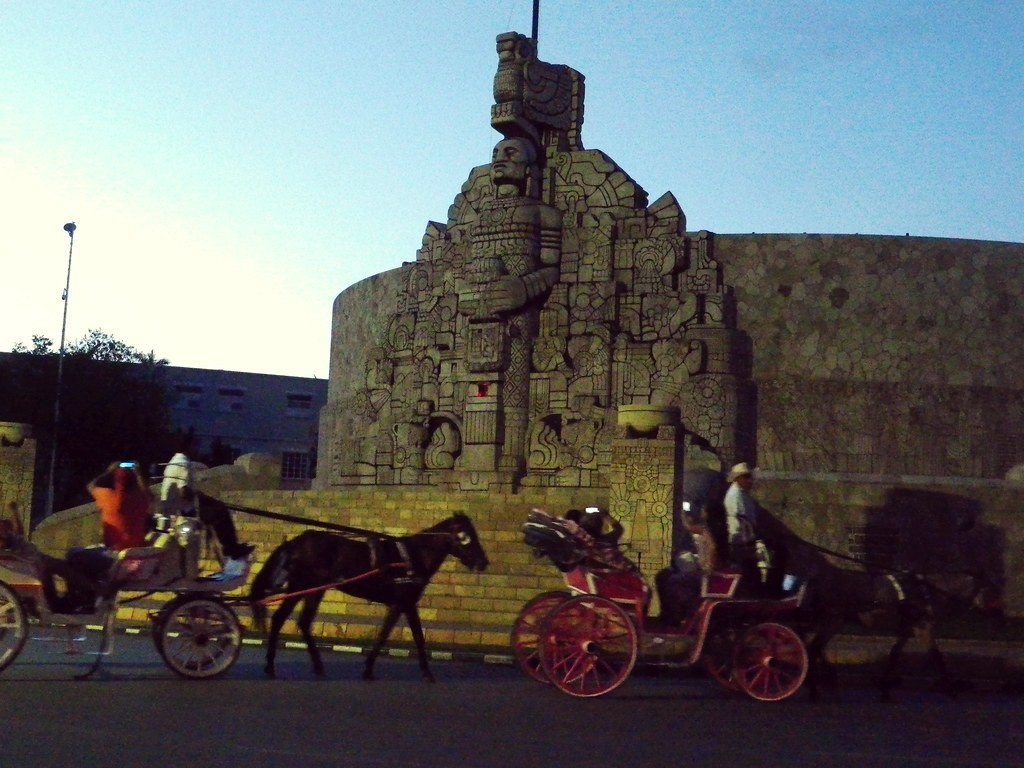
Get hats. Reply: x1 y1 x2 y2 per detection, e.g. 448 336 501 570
727 461 756 483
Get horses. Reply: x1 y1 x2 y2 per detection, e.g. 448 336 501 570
811 563 990 664
246 510 492 685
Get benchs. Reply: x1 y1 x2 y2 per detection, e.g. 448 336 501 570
523 501 648 602
0 564 63 610
87 529 180 589
693 568 741 599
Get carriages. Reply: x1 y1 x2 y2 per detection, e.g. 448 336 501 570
0 486 490 685
510 403 1010 704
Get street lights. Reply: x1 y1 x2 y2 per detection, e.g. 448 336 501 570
43 222 77 517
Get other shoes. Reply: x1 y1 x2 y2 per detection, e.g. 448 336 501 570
654 615 681 628
224 542 253 559
56 599 93 614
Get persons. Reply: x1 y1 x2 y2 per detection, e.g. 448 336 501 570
47 460 155 612
724 462 759 583
160 439 254 558
453 138 560 469
570 507 653 609
655 502 728 625
